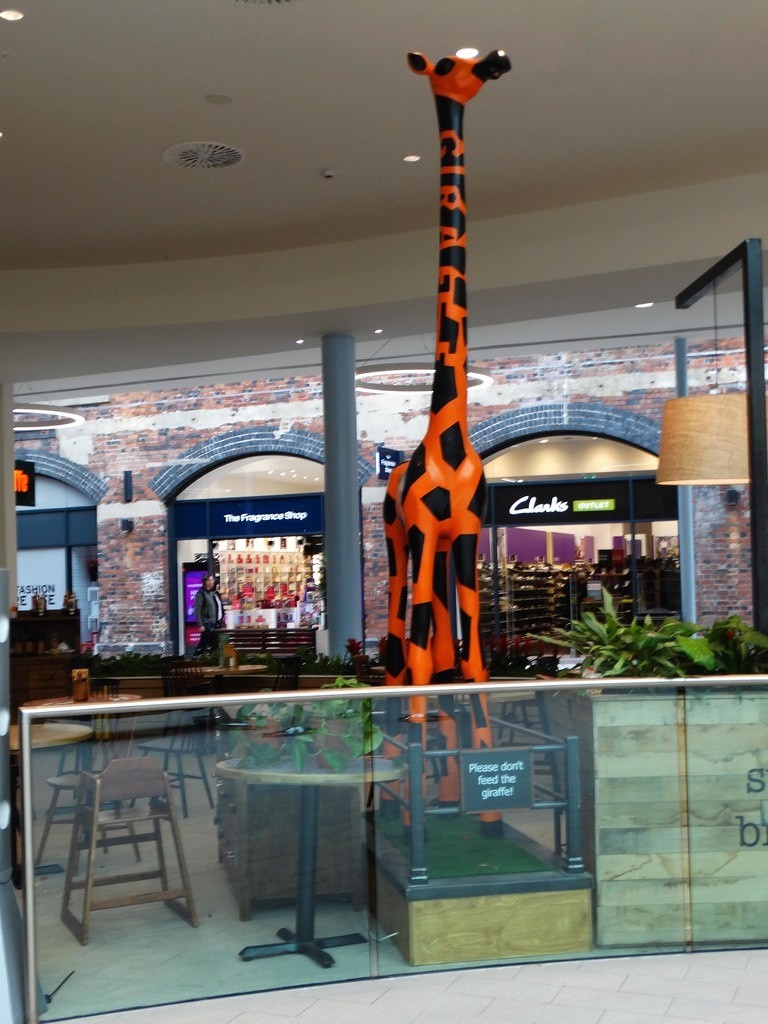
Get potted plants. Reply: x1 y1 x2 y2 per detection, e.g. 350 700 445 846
530 590 768 693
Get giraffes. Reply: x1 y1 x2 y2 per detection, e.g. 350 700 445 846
381 51 513 844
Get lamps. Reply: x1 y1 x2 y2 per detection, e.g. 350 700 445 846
119 520 130 533
656 281 755 486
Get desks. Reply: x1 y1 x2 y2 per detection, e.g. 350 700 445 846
170 663 268 752
18 691 140 773
8 721 91 893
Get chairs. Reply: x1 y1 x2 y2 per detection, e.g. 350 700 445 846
36 656 303 946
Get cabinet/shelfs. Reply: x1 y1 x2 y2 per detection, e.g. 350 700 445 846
477 561 571 641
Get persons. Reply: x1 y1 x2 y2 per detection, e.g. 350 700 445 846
563 572 587 629
194 574 224 656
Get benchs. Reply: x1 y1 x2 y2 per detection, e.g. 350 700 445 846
492 690 538 742
